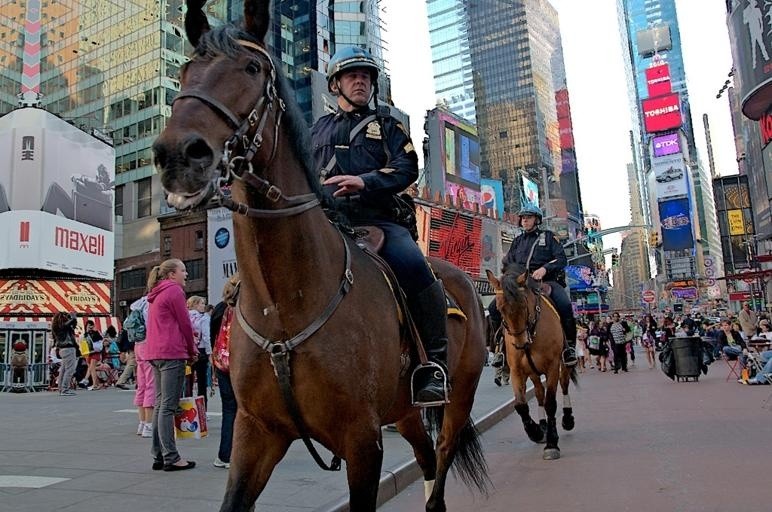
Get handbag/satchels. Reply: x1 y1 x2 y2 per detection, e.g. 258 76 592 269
174 395 209 440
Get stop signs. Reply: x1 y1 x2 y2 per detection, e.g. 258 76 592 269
642 290 655 302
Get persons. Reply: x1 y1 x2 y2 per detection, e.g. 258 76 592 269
51 311 139 391
302 47 457 402
181 295 208 410
641 300 771 385
488 204 578 367
485 297 641 386
209 269 241 469
123 286 155 438
183 296 219 395
143 258 198 471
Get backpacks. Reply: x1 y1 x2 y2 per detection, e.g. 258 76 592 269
123 310 147 342
210 305 236 375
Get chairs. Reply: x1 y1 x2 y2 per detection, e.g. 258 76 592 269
46 348 131 395
717 337 772 387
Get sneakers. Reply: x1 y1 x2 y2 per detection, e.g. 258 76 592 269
212 456 231 469
495 379 502 387
578 362 658 374
745 378 761 384
57 378 129 395
143 425 154 438
136 423 144 435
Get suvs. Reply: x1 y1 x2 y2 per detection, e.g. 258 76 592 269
655 168 683 183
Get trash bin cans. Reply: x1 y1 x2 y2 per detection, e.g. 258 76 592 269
668 336 704 384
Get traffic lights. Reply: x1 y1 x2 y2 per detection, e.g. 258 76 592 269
650 231 659 247
611 254 618 266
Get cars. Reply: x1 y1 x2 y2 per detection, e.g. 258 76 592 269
653 318 720 352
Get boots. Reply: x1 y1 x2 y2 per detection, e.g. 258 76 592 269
560 319 579 366
410 279 451 407
491 320 505 366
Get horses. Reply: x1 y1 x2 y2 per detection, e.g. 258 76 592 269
152 0 496 511
485 268 579 460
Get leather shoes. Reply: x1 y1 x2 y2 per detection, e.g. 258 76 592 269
151 462 164 470
165 461 195 472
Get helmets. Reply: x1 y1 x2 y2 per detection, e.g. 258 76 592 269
517 203 543 218
324 47 379 80
